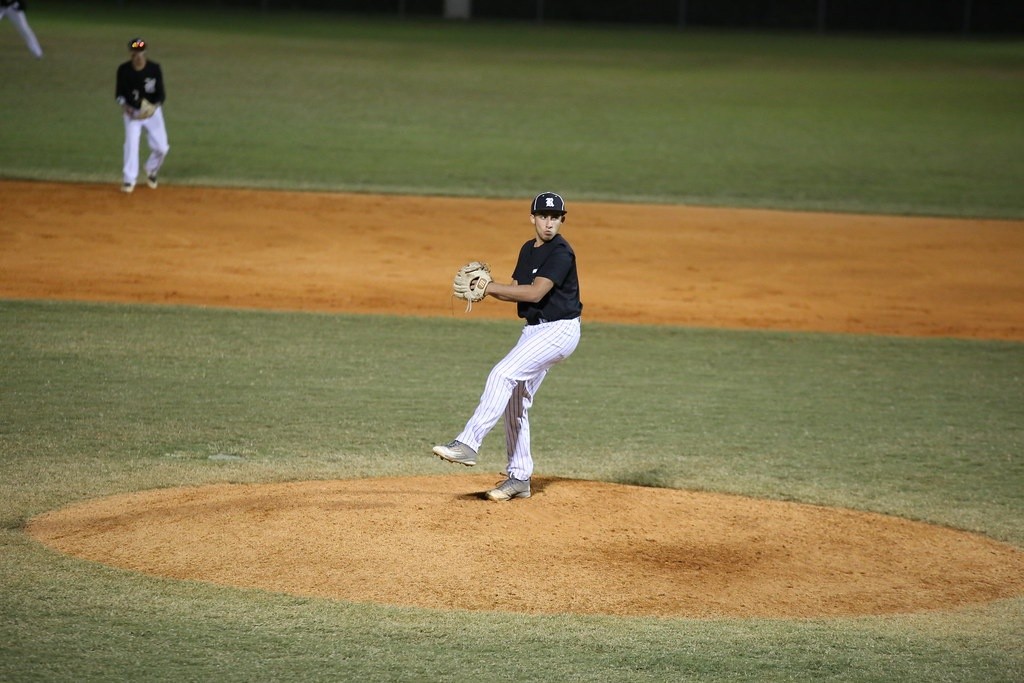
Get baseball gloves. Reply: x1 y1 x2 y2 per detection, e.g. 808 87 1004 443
453 261 492 303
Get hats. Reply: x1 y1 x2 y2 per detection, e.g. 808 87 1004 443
128 38 146 52
531 191 568 216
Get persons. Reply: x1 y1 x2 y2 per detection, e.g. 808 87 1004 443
430 192 584 502
113 38 170 193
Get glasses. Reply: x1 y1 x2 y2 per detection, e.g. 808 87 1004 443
131 41 145 49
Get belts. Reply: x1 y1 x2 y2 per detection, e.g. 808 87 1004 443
526 317 559 325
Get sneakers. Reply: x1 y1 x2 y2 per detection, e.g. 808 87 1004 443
147 175 158 190
121 182 136 192
485 477 532 501
432 440 477 466
